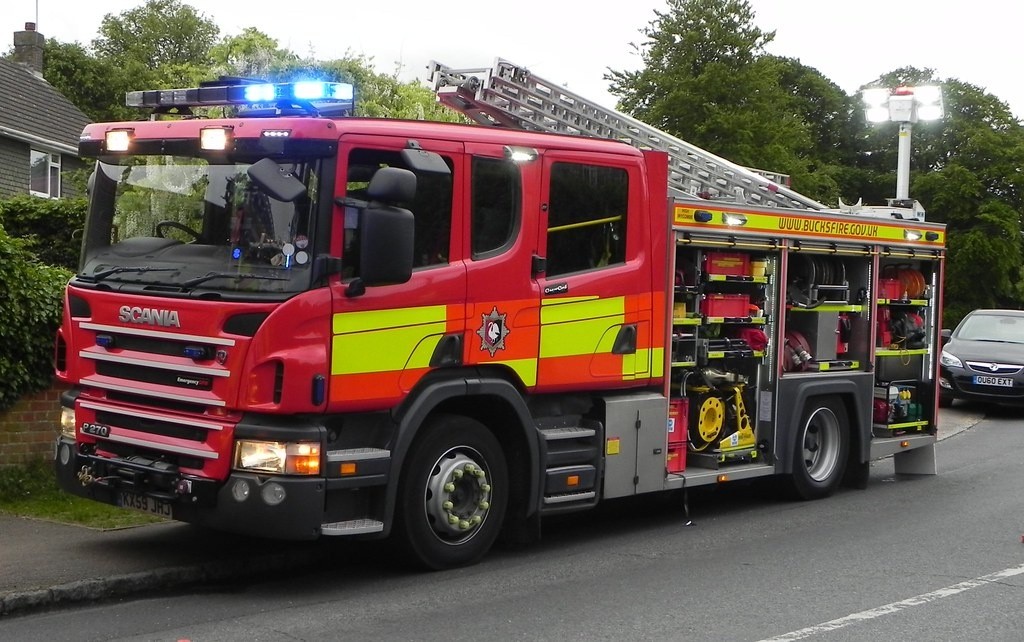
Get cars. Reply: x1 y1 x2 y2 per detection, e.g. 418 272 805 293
938 308 1024 412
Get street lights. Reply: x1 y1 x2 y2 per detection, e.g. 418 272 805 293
864 84 945 199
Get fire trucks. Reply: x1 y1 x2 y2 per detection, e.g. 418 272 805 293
55 55 951 572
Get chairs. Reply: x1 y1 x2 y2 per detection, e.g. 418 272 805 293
236 173 314 247
